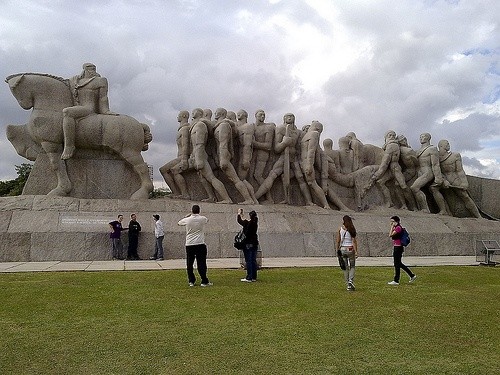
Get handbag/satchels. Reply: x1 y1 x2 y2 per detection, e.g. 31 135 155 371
337 249 346 270
234 232 248 250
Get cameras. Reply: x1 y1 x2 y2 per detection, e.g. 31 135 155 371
240 209 243 213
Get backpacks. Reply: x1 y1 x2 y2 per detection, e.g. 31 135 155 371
394 225 410 246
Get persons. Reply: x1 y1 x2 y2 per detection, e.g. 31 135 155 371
387 216 416 285
237 208 258 283
177 204 213 287
159 108 487 217
336 215 359 290
149 214 165 261
127 213 144 261
59 62 120 159
109 215 126 261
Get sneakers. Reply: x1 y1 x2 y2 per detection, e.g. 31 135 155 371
409 276 416 282
388 281 400 285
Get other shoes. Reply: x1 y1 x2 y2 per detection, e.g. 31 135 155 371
252 279 256 281
349 282 355 290
112 258 117 261
189 282 196 286
135 258 143 260
150 257 157 260
241 279 252 283
346 287 352 290
201 283 213 287
156 259 164 261
126 258 134 260
117 258 124 260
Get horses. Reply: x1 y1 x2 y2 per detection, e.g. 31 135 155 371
5 71 155 200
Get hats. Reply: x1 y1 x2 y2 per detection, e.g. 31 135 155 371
153 215 159 220
